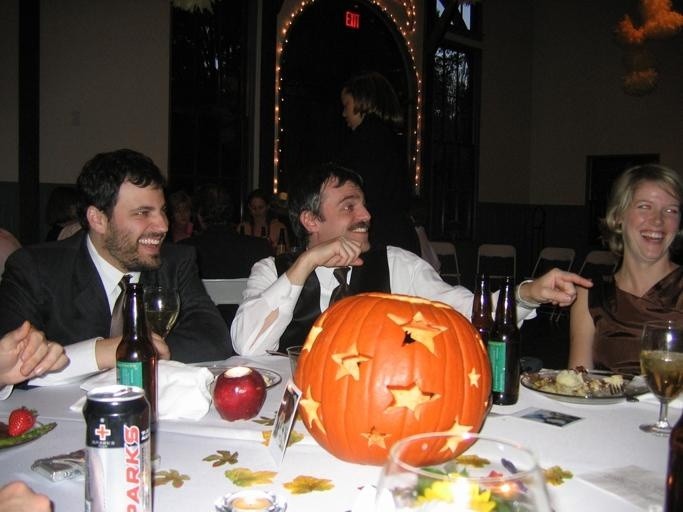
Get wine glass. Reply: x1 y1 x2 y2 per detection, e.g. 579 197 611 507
142 285 180 341
638 319 683 437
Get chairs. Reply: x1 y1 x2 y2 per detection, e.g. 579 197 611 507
523 246 575 319
198 278 250 311
555 250 620 322
476 244 516 291
431 242 461 286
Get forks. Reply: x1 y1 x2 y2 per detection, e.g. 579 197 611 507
609 383 639 402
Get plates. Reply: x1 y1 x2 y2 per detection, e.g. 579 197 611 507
205 366 282 389
520 370 650 405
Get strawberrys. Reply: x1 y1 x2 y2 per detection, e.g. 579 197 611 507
5 405 38 437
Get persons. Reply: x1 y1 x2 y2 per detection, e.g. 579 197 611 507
0 148 235 387
0 318 71 392
568 163 683 373
164 181 299 324
228 160 596 362
1 480 51 512
2 183 83 271
297 69 419 258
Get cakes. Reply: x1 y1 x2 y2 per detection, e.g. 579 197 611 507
526 368 627 396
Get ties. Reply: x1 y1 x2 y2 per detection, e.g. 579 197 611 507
329 268 354 306
109 277 132 336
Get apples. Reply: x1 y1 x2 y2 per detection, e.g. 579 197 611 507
214 366 267 422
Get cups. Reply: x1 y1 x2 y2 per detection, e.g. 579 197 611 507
372 432 554 512
285 345 304 377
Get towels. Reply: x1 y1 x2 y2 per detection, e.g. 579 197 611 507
70 357 214 422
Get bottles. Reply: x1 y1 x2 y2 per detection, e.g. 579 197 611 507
114 283 157 461
470 274 495 347
485 276 520 405
274 228 286 257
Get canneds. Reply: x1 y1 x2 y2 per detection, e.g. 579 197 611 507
83 384 152 512
